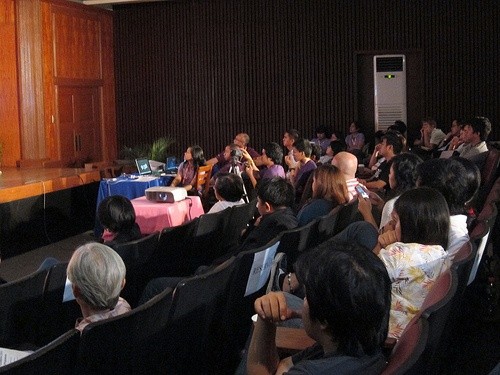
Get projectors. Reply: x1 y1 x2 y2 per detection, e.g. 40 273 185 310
145 186 187 202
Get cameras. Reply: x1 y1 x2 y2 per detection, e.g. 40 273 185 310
229 149 244 166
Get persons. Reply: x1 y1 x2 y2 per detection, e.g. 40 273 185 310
139 176 300 308
41 195 142 270
0 244 131 366
171 116 491 227
265 157 480 295
237 188 451 375
246 242 392 375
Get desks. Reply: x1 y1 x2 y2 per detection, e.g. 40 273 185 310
101 196 204 243
93 173 162 237
131 165 180 187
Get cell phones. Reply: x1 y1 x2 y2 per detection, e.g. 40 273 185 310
354 185 369 199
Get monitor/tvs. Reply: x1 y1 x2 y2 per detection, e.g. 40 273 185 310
134 157 152 175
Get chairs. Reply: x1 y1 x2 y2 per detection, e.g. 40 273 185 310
0 142 500 375
